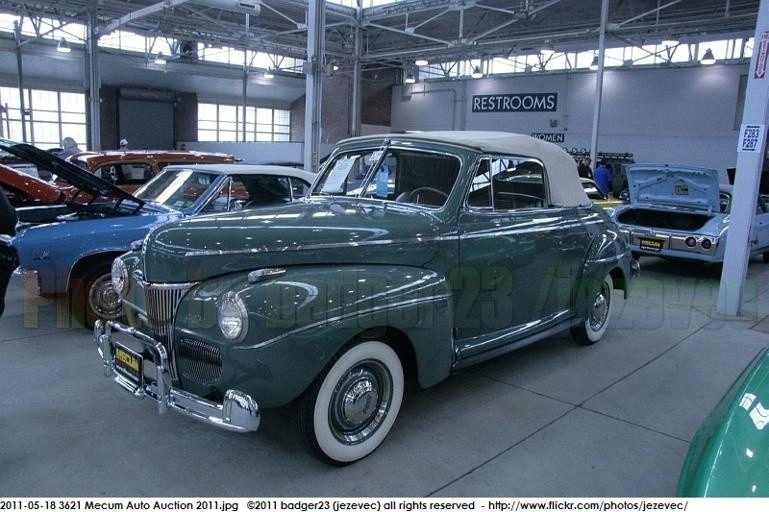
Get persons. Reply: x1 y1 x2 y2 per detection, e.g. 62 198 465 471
594 162 614 200
611 176 629 201
578 158 594 190
0 184 22 318
118 138 135 180
55 136 84 161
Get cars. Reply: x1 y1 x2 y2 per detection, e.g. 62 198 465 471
89 129 636 470
575 162 769 279
0 136 319 335
